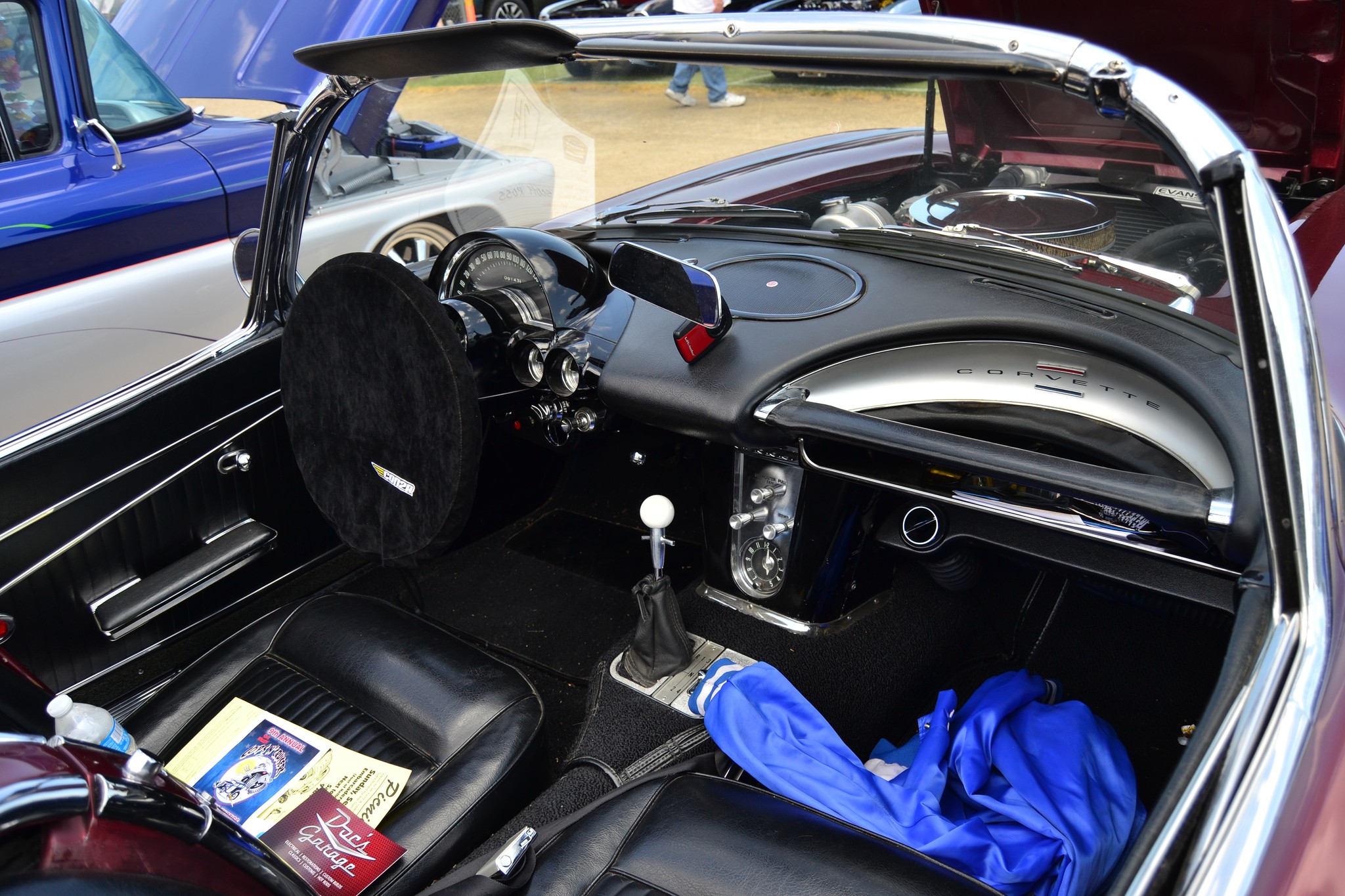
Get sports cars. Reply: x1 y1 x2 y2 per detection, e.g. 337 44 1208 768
1 10 1345 896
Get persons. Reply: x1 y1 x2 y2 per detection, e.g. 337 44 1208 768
664 0 746 108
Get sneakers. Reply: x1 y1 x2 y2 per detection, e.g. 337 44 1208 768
665 88 697 106
708 92 746 107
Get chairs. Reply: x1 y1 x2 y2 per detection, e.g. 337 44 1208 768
523 769 1007 896
0 593 549 896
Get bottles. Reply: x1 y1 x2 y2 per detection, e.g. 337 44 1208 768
45 695 168 786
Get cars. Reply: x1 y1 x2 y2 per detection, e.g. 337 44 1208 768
476 0 912 78
0 0 449 300
